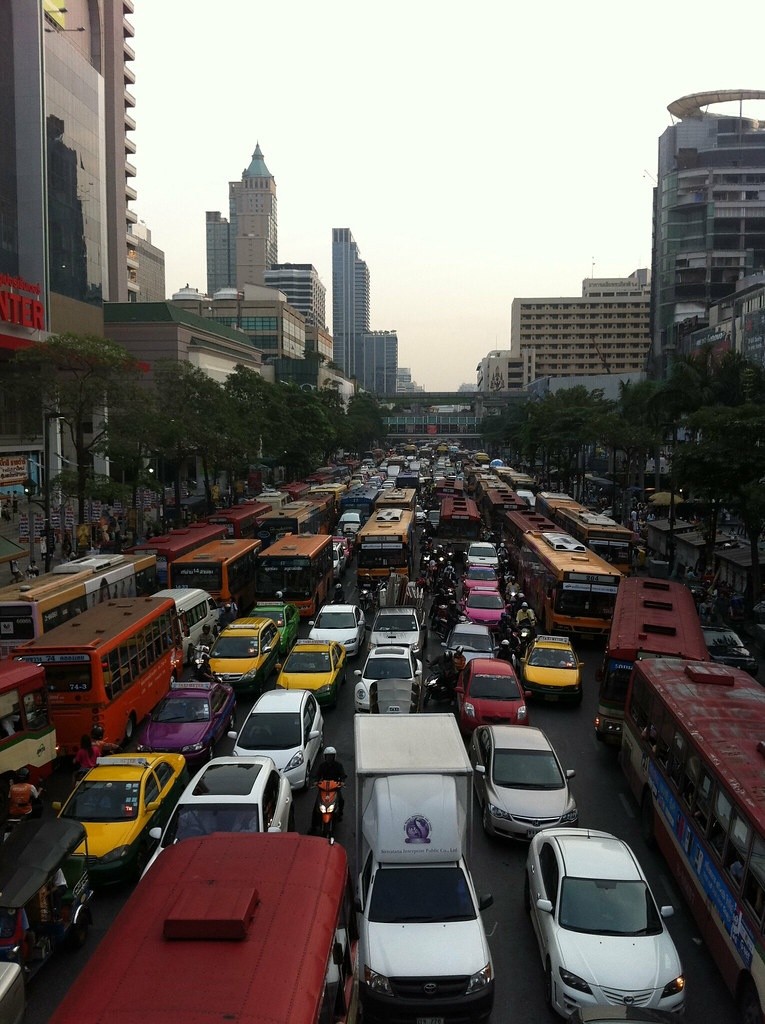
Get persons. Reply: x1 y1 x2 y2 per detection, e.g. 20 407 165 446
326 499 342 524
644 723 751 887
632 503 745 629
274 525 537 835
539 471 656 532
21 859 68 973
189 596 238 669
82 787 114 811
212 486 261 515
417 465 435 511
0 470 209 582
7 765 45 818
74 724 124 770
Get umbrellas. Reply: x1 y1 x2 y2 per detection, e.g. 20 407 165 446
649 492 686 506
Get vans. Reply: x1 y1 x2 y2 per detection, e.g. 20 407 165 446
149 587 223 670
208 617 282 696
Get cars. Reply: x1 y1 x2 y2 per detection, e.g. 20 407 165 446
275 638 348 710
352 646 424 713
52 752 189 882
519 635 586 710
467 724 579 846
227 688 325 794
453 658 532 734
700 624 759 677
138 754 294 885
134 680 238 766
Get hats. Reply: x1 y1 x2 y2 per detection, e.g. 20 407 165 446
511 576 516 580
518 593 525 600
335 583 342 590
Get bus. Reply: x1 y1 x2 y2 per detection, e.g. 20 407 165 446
620 656 765 1024
0 551 159 660
166 538 264 619
0 659 60 812
4 597 184 757
47 833 355 1024
595 576 710 736
206 440 638 675
126 521 229 592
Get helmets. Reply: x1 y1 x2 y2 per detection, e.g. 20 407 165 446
203 647 209 652
506 628 512 634
444 650 453 662
456 646 464 656
522 602 528 607
501 612 506 619
501 640 509 650
276 591 282 599
506 615 511 620
202 624 210 633
323 746 337 761
225 604 231 612
449 599 457 605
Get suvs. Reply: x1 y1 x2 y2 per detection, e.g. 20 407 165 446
524 827 687 1024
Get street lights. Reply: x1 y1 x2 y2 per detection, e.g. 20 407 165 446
23 412 72 574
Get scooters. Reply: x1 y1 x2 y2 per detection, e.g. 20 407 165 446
309 773 349 838
73 738 124 789
8 776 48 818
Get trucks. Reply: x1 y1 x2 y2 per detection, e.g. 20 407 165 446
347 711 496 1024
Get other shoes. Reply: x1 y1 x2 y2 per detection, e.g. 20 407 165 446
450 698 455 706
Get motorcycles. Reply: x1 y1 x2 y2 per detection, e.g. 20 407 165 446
0 817 94 987
